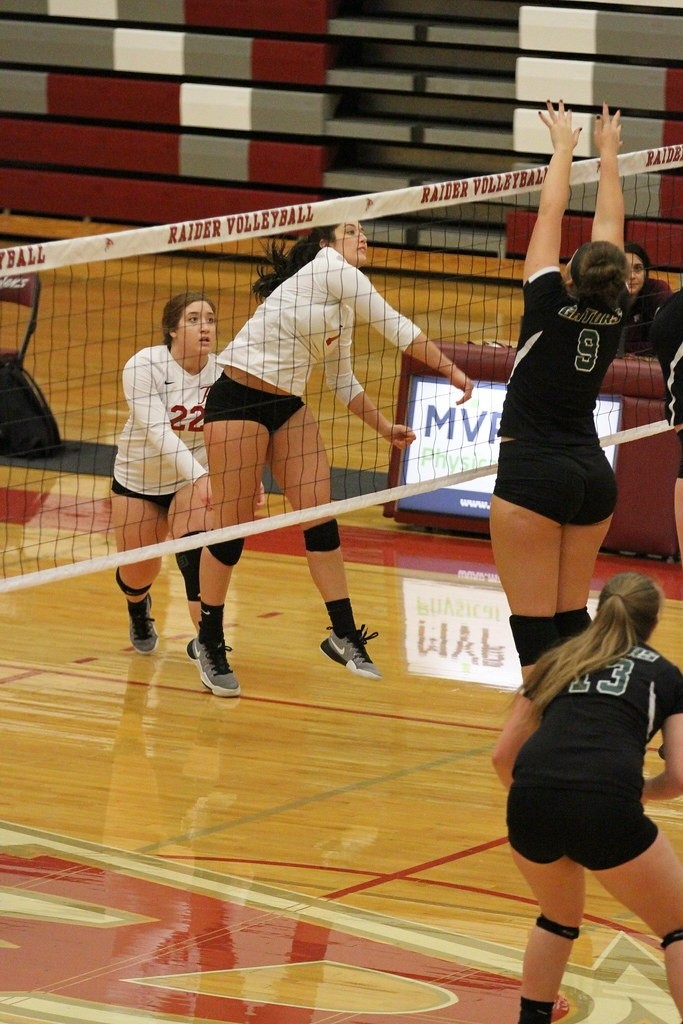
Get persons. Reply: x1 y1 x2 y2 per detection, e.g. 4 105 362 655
489 100 683 685
491 573 683 1024
111 291 267 655
187 220 474 697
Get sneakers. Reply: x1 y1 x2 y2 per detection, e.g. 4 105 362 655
127 590 159 655
319 624 383 681
186 621 241 697
